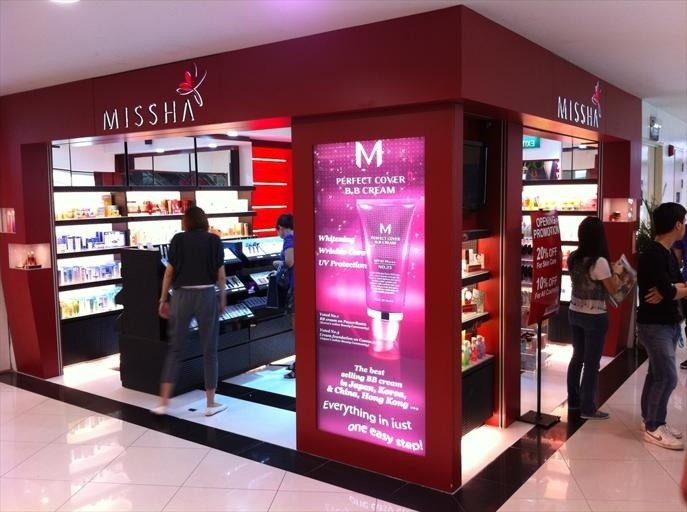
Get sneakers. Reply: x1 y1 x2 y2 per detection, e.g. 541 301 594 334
204 403 227 416
151 406 167 415
640 420 684 450
580 411 609 420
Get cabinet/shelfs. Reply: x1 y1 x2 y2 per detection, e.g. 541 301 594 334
521 179 599 373
462 271 496 438
54 186 257 368
114 236 295 399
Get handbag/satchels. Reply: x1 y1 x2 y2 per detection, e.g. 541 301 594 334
265 269 288 309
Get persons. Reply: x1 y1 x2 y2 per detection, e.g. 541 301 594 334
272 214 296 379
151 207 229 416
635 202 687 450
673 224 687 369
566 216 625 420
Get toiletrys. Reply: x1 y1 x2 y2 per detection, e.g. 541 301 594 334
356 198 417 352
461 334 485 366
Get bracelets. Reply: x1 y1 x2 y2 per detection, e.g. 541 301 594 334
612 272 621 279
